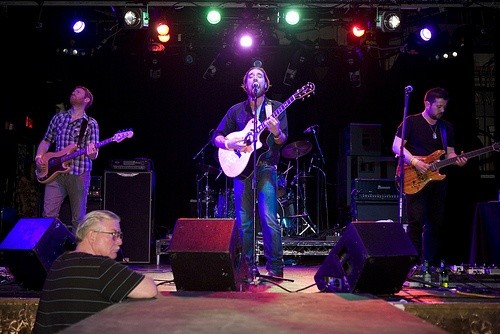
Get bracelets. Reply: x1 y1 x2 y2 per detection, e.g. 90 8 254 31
225 140 233 151
410 158 419 167
273 129 283 141
35 155 41 161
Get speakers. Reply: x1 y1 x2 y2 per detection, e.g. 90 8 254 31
168 217 251 293
103 170 152 263
471 201 500 269
58 197 102 231
354 201 400 222
0 217 78 292
313 221 418 295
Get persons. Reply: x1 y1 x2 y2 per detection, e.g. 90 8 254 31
35 85 99 236
212 66 289 281
31 210 158 334
393 88 467 273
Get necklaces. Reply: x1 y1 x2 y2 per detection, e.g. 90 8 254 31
422 112 438 139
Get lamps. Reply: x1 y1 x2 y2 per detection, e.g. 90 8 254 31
66 0 440 57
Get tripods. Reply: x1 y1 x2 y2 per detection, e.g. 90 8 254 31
248 92 318 287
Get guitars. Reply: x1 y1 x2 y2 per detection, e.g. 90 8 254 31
217 81 316 182
35 128 134 184
396 142 500 196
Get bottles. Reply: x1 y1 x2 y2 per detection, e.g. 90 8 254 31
440 261 449 288
422 260 432 287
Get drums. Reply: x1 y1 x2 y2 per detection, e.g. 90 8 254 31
214 188 236 218
253 197 285 238
275 170 287 199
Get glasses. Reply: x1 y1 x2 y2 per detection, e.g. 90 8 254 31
94 231 124 241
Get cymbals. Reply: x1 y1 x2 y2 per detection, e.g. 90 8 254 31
213 149 220 165
304 124 318 134
298 175 316 179
281 140 313 159
193 162 215 174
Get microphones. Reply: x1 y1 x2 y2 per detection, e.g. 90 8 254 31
405 86 413 92
304 126 316 134
291 172 301 185
308 158 313 172
253 82 259 94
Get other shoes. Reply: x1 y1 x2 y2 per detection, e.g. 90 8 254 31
264 273 282 282
